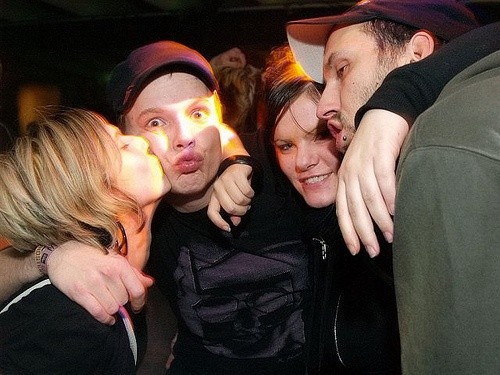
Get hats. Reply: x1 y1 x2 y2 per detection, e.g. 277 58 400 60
287 0 479 84
104 40 219 116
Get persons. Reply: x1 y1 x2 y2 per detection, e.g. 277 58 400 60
0 40 320 375
260 22 500 375
0 105 254 375
286 0 500 374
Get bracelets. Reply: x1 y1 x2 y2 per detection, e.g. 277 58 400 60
219 154 258 176
35 243 58 274
